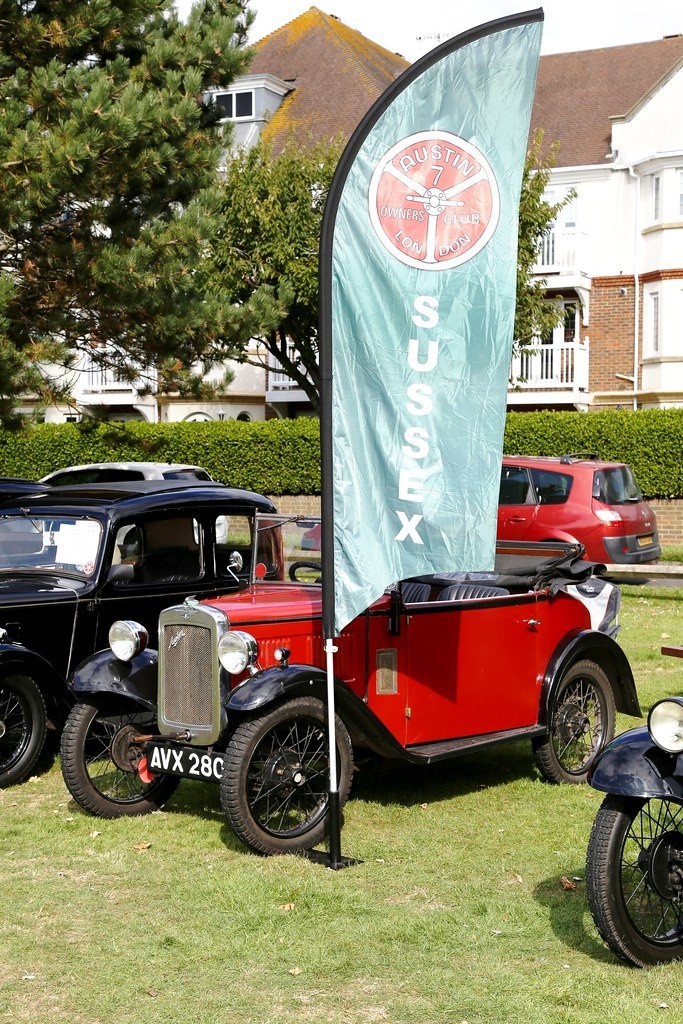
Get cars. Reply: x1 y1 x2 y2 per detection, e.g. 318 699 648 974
584 644 683 970
57 510 642 857
0 478 288 792
0 459 231 590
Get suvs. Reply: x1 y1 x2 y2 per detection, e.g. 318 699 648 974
299 452 664 584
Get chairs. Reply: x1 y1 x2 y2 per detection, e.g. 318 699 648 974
498 478 523 504
536 472 565 501
398 582 511 604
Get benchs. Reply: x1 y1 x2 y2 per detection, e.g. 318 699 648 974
136 545 273 584
